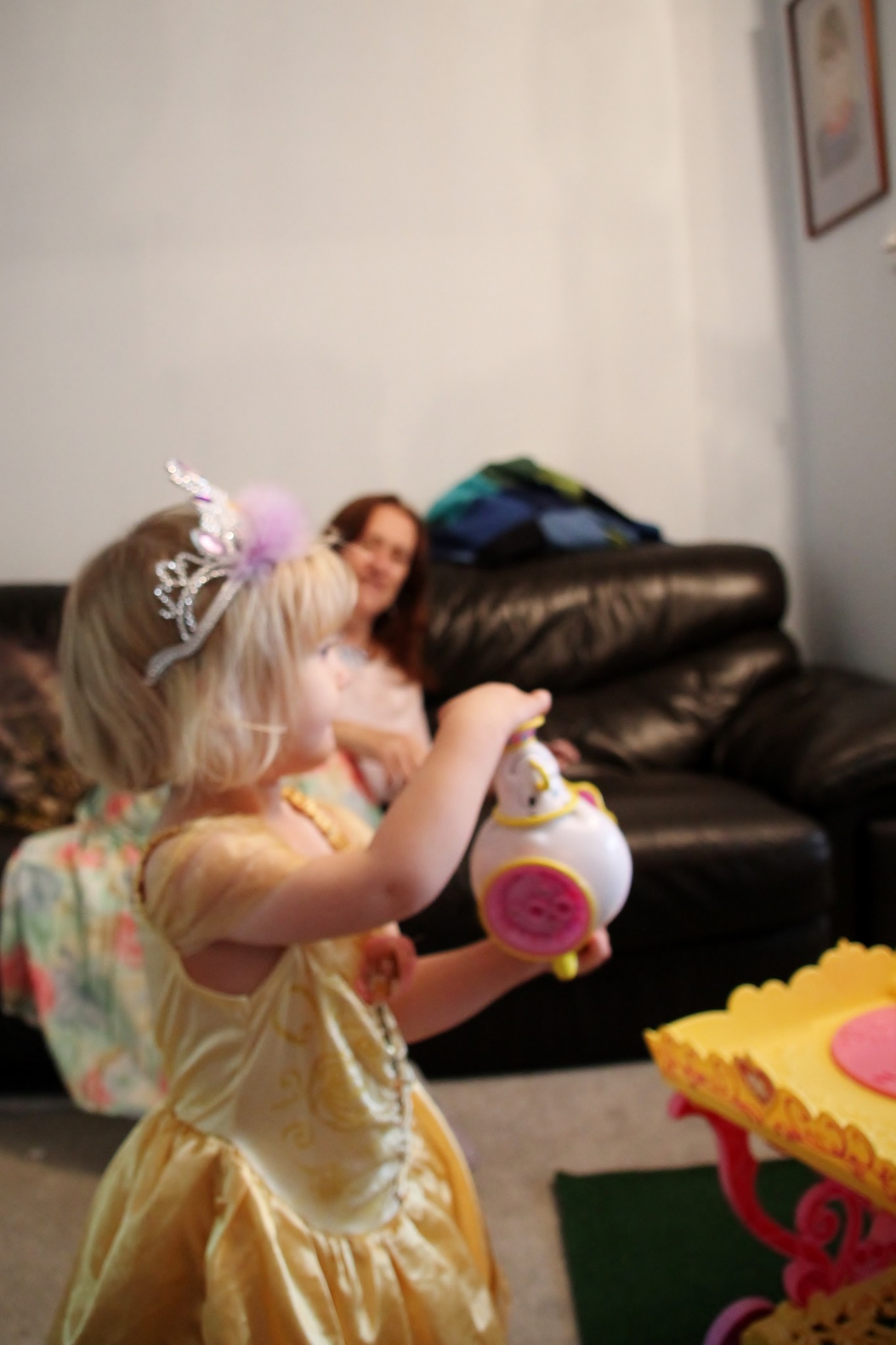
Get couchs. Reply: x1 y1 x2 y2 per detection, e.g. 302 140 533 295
1 545 896 1083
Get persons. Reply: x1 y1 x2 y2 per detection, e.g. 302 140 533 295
53 492 610 1344
812 6 863 180
5 492 431 1110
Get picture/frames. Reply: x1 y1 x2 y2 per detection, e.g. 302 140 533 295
786 1 889 241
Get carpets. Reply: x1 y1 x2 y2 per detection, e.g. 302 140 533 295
551 1157 826 1345
